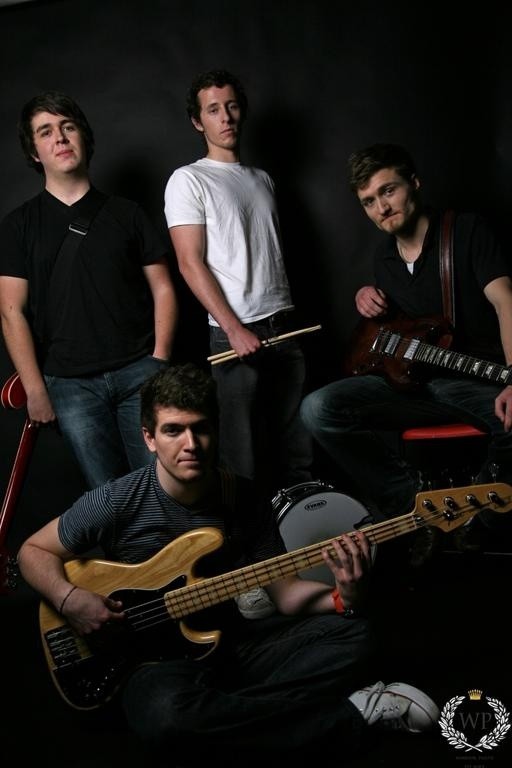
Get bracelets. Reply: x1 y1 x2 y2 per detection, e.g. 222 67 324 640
51 582 80 617
330 588 356 622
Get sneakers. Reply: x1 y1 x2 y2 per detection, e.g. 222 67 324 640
453 526 480 550
236 586 275 619
349 681 439 733
409 528 434 565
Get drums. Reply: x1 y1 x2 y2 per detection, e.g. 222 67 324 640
271 480 376 580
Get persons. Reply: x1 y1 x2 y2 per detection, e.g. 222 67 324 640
0 90 180 494
13 364 440 760
162 67 319 618
296 141 510 508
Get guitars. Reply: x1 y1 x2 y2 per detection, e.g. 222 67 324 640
37 481 510 709
351 321 511 385
1 374 39 595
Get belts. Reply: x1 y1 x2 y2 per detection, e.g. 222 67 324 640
253 311 295 329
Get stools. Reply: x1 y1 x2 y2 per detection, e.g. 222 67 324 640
403 424 484 441
